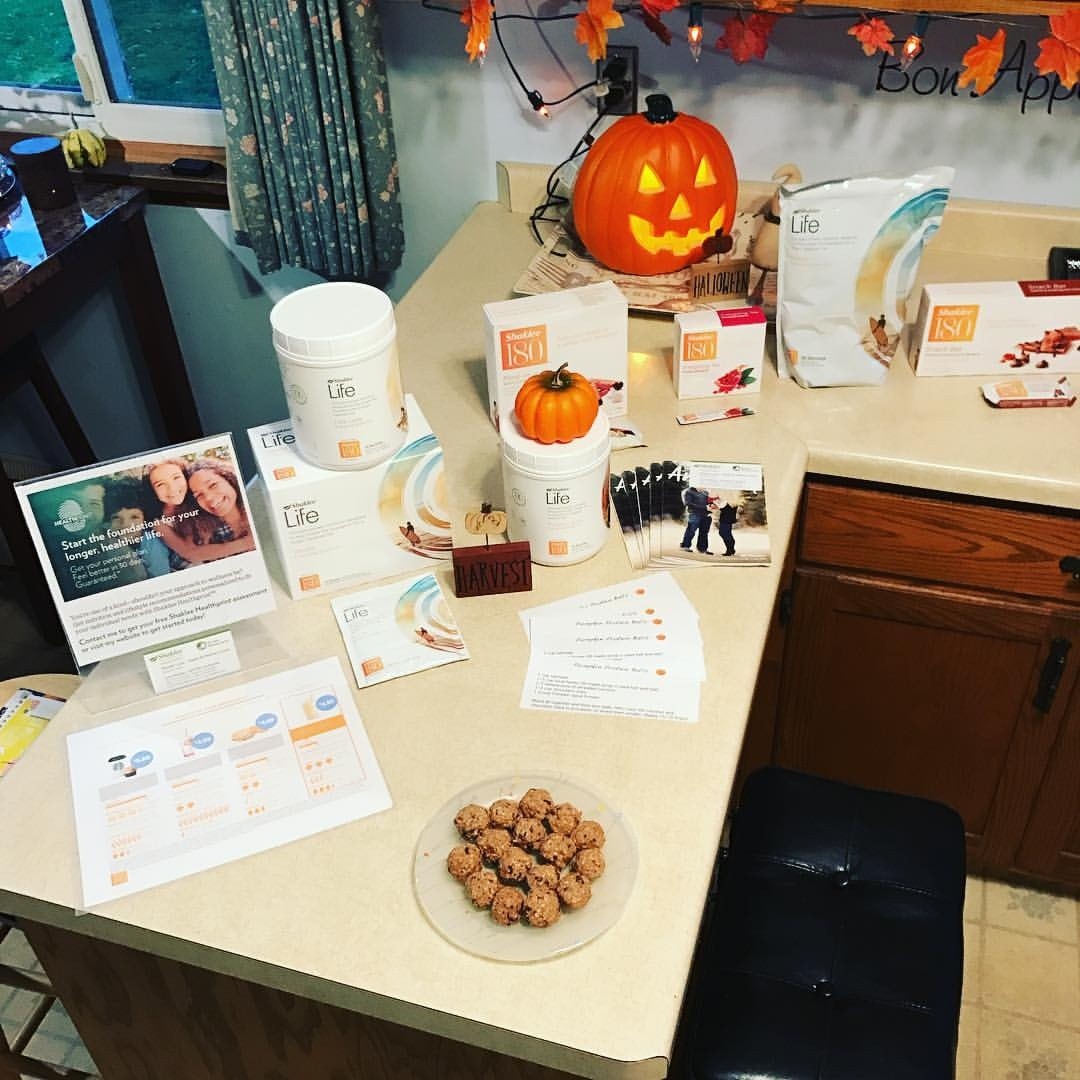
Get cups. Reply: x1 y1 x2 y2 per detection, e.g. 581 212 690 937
9 136 74 211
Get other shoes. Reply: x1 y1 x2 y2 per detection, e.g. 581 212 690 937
701 551 713 555
682 547 693 552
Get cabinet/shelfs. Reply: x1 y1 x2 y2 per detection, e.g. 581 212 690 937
770 474 1080 901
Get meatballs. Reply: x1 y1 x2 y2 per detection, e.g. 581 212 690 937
446 787 604 930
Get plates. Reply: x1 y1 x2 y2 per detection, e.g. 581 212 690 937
0 167 16 199
511 212 777 327
411 775 639 962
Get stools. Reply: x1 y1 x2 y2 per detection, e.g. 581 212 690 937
1 665 90 786
669 759 972 1080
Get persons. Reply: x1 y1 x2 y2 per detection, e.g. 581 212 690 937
50 460 254 599
679 485 718 555
714 495 739 556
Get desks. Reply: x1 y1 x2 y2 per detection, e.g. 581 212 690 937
0 174 204 489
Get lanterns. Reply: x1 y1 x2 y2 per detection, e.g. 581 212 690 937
575 94 738 276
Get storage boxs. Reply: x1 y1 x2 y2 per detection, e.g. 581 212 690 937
497 405 615 572
1046 244 1080 279
476 276 631 430
249 395 466 600
904 277 1080 379
675 302 770 403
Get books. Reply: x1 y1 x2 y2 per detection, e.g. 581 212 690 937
609 457 773 573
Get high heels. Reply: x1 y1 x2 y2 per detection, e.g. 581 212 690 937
722 550 735 556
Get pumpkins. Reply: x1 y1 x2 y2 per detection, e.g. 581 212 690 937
62 112 105 169
514 362 599 444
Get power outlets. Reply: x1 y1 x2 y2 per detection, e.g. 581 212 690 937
590 46 645 115
